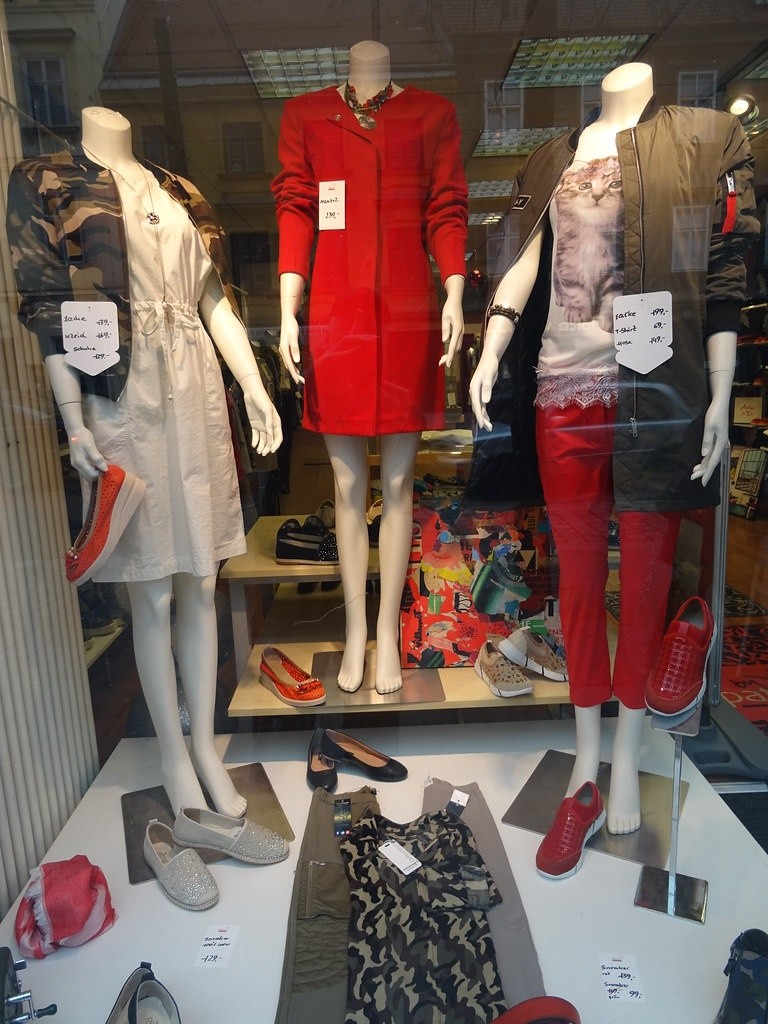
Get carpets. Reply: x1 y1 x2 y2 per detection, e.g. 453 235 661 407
605 582 768 624
721 624 768 736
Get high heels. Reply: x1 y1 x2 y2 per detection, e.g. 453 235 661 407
66 465 147 585
259 646 326 707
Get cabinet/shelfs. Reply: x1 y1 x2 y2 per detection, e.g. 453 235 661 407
220 516 622 730
61 443 131 670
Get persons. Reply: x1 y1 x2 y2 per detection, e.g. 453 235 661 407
463 62 740 836
269 42 468 699
17 105 285 827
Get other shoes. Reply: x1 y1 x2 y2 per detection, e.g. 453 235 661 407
274 498 384 565
475 641 535 698
535 782 607 880
171 806 289 864
307 728 337 792
79 603 127 650
104 962 181 1024
737 334 768 450
320 729 408 782
500 627 569 681
297 581 340 593
643 596 718 716
143 818 219 911
